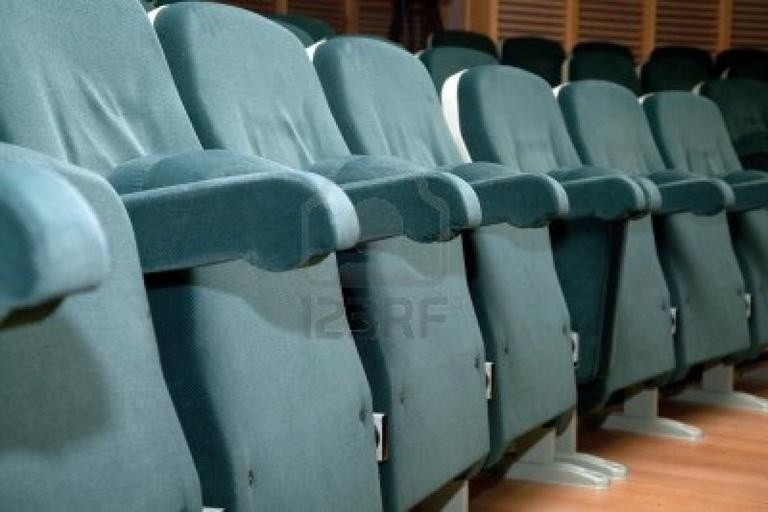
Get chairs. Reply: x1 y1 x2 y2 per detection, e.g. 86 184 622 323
0 0 768 512
